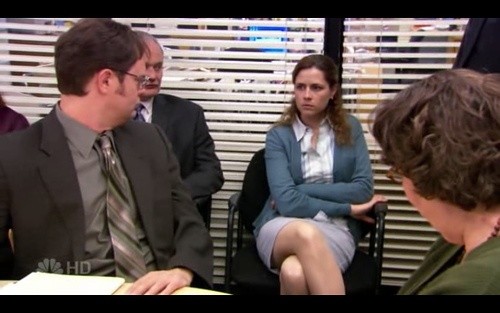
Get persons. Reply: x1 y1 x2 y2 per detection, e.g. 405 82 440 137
253 54 388 295
367 69 500 295
0 18 224 295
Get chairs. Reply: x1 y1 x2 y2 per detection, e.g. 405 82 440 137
225 148 387 294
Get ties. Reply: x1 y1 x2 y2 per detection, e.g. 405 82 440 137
99 134 149 283
134 103 146 122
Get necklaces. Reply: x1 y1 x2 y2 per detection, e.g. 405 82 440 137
486 218 500 240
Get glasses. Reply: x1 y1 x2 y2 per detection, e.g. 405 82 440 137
96 65 149 89
